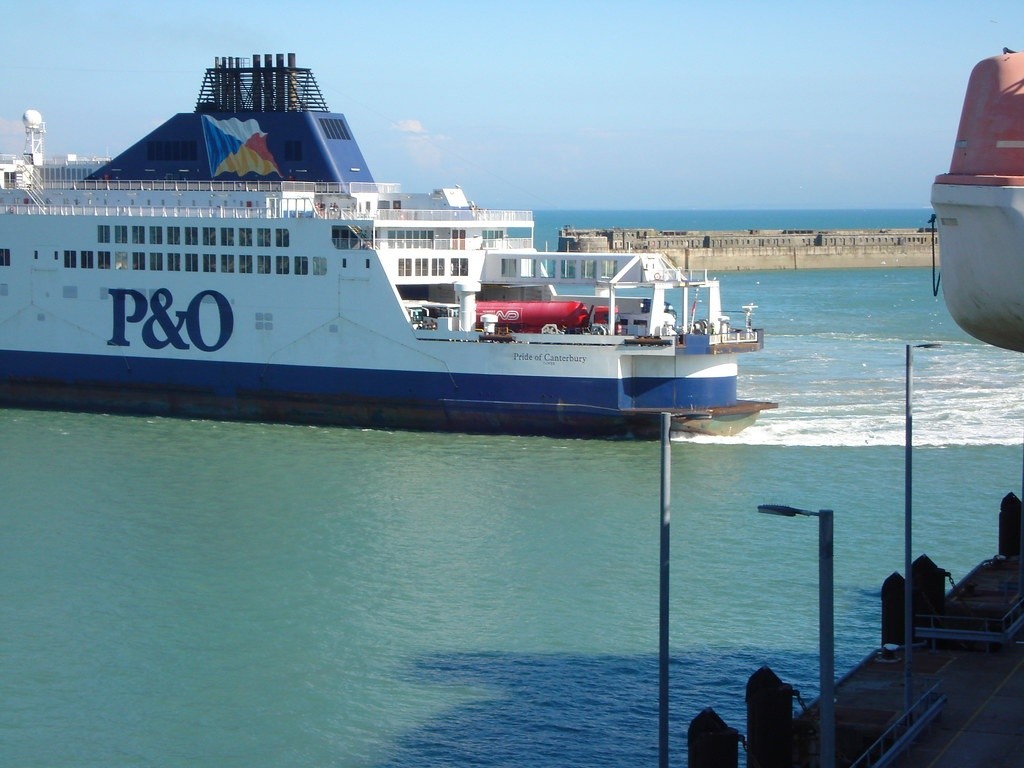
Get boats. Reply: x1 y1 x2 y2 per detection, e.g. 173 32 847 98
1 51 780 439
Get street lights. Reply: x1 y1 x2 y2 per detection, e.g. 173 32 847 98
905 344 945 718
758 503 838 768
658 411 711 768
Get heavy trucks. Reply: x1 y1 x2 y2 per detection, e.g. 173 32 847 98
476 299 621 337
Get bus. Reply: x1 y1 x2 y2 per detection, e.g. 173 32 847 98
422 302 460 326
402 300 426 331
